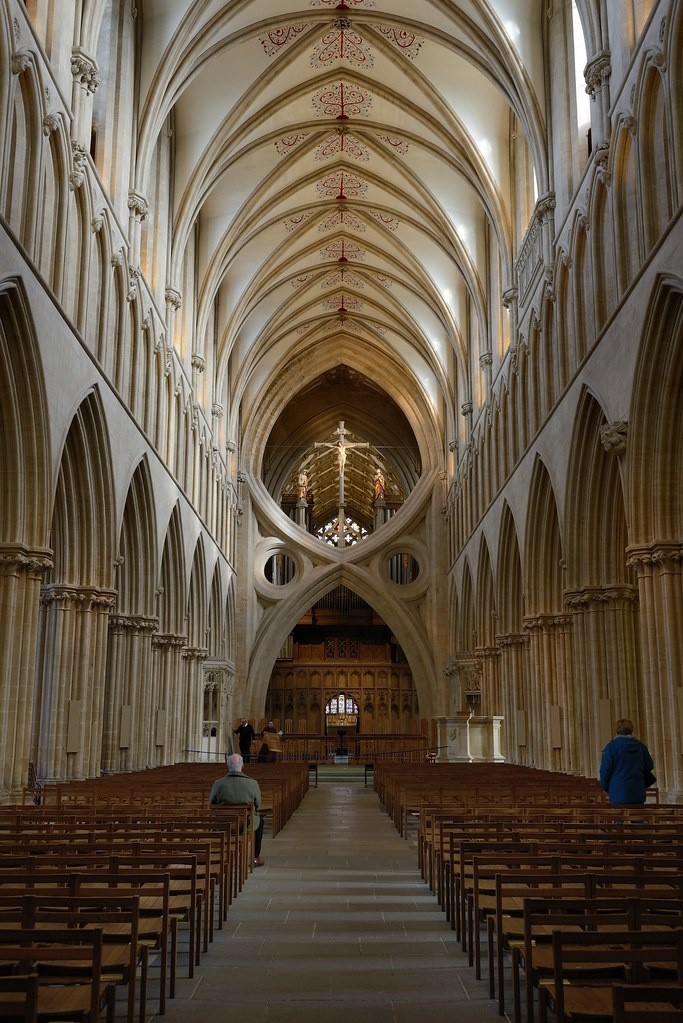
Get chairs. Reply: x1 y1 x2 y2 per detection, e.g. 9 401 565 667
0 762 308 1023
373 760 683 1023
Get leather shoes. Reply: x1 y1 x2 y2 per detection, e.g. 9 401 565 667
254 857 265 866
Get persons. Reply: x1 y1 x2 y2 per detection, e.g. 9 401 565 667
234 717 257 764
261 720 278 738
299 470 309 499
600 718 655 823
209 753 266 868
325 440 360 478
374 468 387 500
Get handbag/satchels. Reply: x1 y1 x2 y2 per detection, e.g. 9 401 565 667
641 742 657 788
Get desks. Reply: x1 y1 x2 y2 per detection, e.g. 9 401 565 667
308 763 317 788
364 764 374 788
